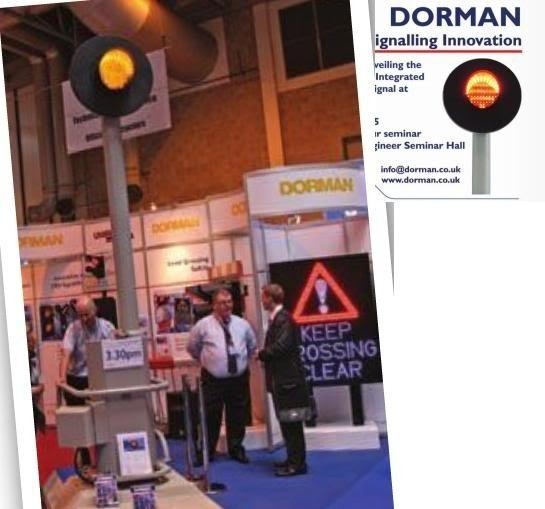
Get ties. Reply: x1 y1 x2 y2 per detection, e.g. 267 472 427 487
222 323 237 373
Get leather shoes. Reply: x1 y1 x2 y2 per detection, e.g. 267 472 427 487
236 454 248 464
194 459 202 467
275 467 307 476
274 460 289 468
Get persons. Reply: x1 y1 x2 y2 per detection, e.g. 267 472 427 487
185 288 258 469
51 296 129 469
254 282 319 477
